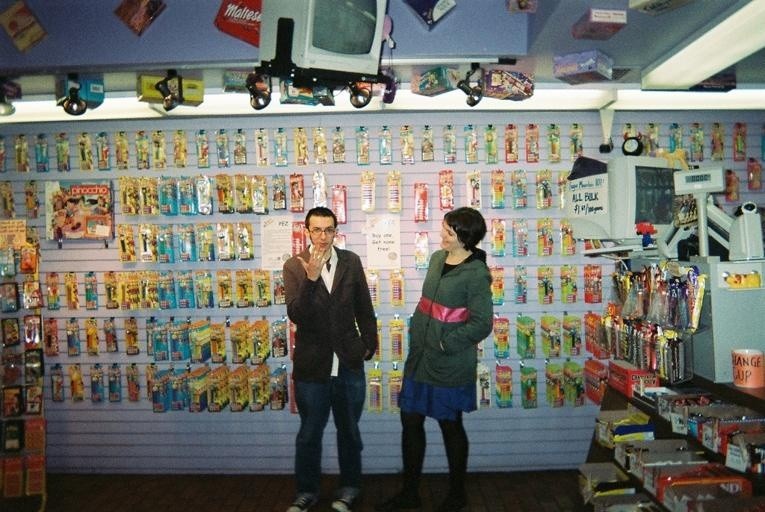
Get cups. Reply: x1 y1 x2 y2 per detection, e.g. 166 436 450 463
731 348 765 389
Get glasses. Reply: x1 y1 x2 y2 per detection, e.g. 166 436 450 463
309 227 334 234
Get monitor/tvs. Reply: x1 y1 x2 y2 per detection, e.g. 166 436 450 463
566 156 694 246
260 0 388 76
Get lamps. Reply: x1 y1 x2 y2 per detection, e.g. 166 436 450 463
156 68 185 111
457 62 483 107
1 74 18 115
246 73 271 110
347 80 373 108
63 73 88 116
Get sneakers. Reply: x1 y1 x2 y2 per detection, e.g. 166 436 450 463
286 495 317 512
438 494 468 512
332 494 357 512
376 493 421 512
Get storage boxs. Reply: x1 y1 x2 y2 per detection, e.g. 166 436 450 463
577 360 765 512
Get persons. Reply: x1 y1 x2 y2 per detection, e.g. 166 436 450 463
280 206 380 512
374 207 495 512
79 195 110 214
